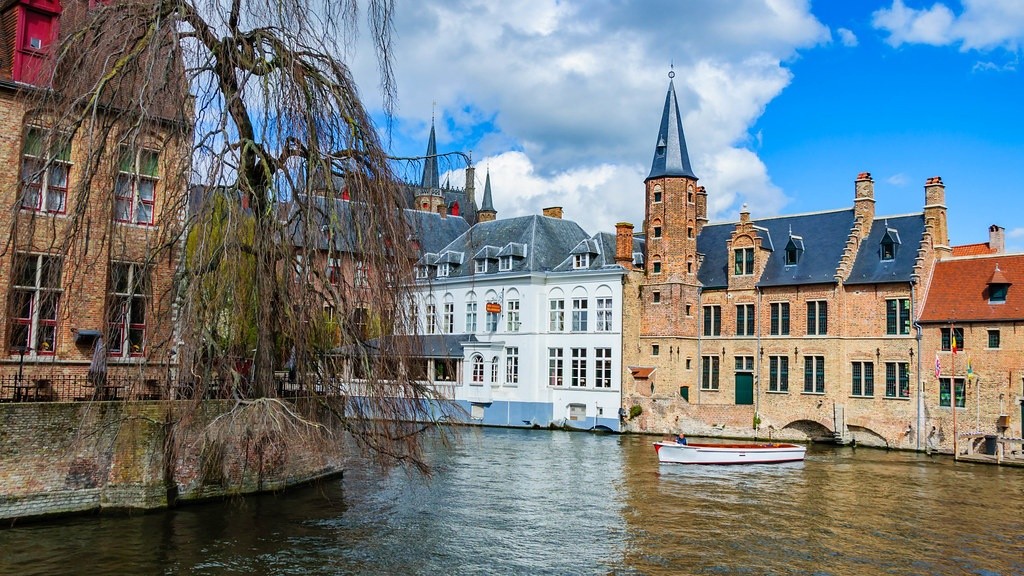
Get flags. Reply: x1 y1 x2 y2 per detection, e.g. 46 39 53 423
951 325 958 354
934 354 942 380
966 352 974 379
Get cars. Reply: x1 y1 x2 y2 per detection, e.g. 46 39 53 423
206 358 252 397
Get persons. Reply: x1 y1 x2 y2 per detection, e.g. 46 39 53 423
678 433 687 447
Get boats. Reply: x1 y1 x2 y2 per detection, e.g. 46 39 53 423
652 440 808 465
